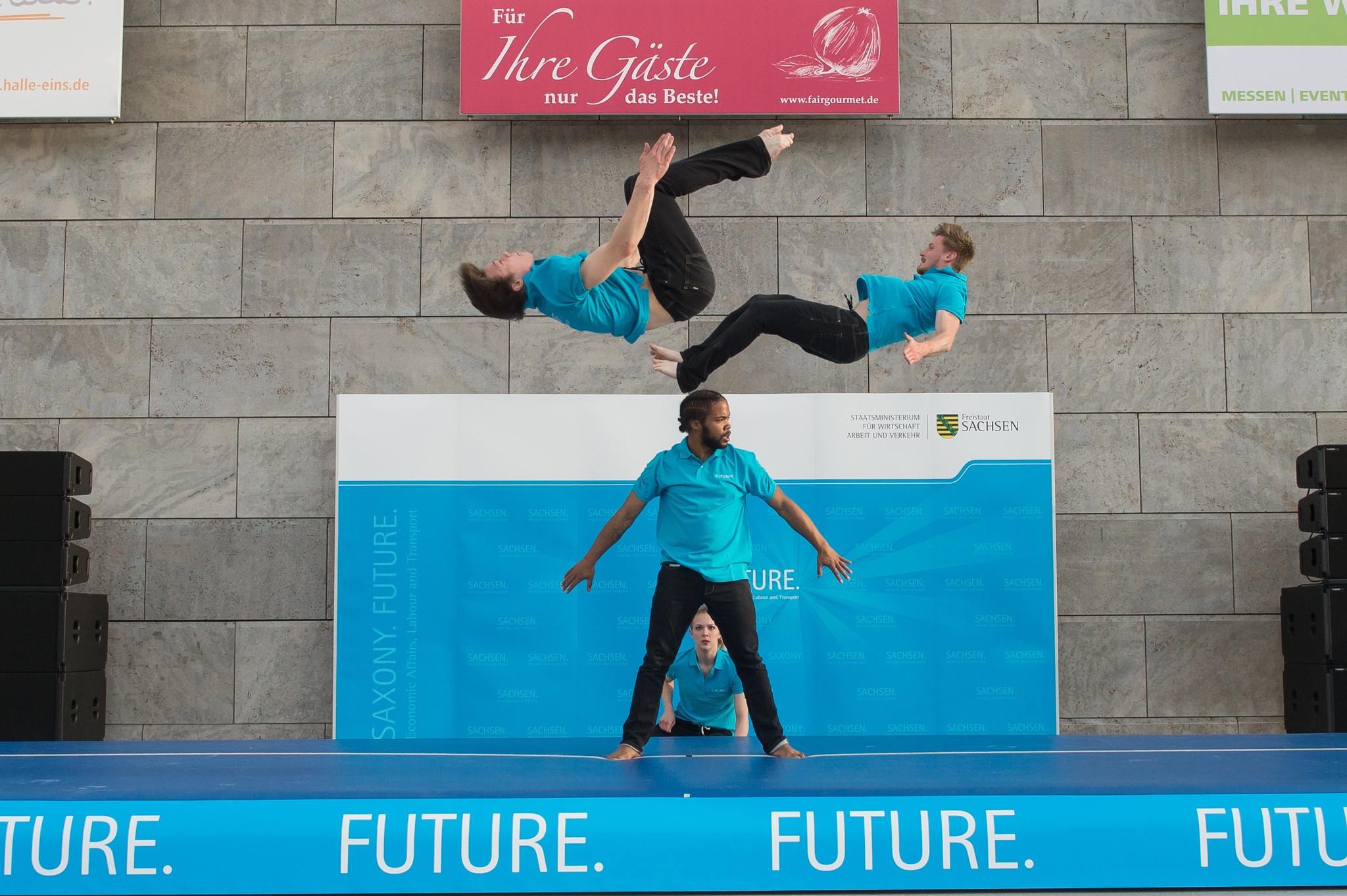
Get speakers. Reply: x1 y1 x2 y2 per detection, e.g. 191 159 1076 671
1299 533 1347 579
0 672 107 741
1282 664 1347 733
1295 444 1347 488
0 496 92 542
1298 489 1347 534
1280 583 1347 665
0 450 92 496
0 541 90 592
0 592 109 672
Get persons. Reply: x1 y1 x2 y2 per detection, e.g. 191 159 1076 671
650 222 976 394
650 606 749 736
459 124 795 344
561 390 853 761
